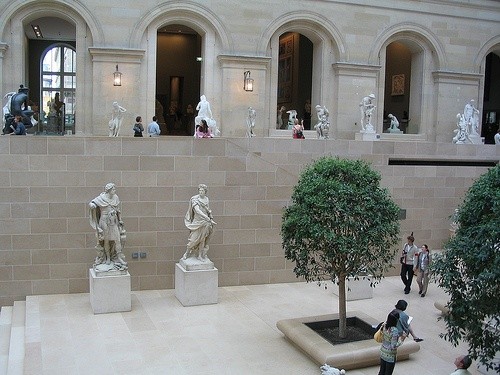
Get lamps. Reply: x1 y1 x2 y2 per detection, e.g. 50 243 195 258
244 71 254 92
113 64 122 86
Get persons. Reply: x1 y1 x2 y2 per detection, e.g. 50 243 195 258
47 98 57 122
132 116 145 137
4 85 38 133
391 300 424 342
11 115 25 134
400 235 418 294
182 182 216 260
246 94 498 145
88 183 127 266
434 301 453 319
416 244 431 296
196 95 217 134
494 126 500 144
195 119 213 138
292 120 305 139
448 355 474 375
148 115 160 137
108 101 126 137
378 311 399 375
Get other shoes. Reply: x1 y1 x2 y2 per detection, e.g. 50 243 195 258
414 338 423 342
421 294 425 297
419 290 421 294
404 287 409 294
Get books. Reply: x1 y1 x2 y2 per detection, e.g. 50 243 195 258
407 315 414 325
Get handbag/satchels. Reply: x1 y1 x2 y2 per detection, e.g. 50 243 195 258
374 323 384 343
